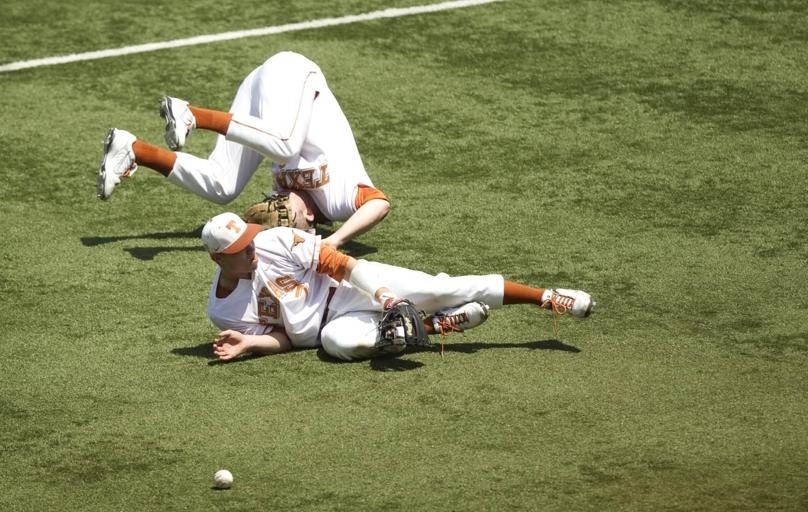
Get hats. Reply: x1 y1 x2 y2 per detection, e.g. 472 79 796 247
201 212 261 255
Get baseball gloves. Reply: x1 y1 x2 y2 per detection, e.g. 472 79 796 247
375 299 432 354
244 195 289 227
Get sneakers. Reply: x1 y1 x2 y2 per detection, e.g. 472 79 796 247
540 288 593 319
157 94 198 152
97 127 137 200
431 301 489 334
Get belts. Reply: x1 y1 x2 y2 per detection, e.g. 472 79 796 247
320 287 336 330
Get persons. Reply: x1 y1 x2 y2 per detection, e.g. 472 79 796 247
95 52 393 254
201 212 595 365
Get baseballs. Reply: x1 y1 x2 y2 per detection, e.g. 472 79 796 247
215 470 233 488
